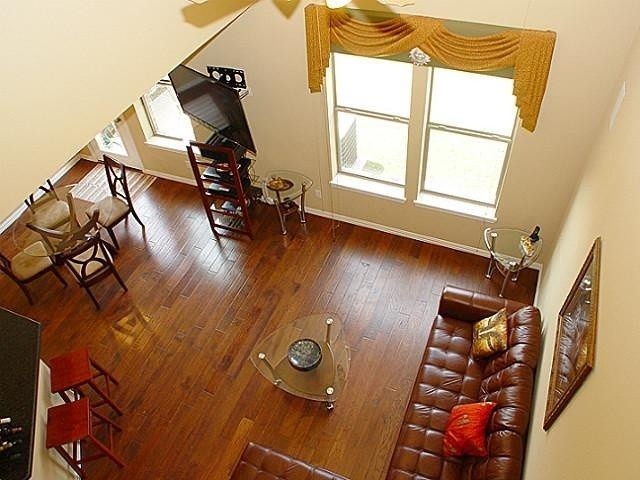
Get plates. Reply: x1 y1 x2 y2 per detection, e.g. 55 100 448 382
267 178 294 191
288 337 322 372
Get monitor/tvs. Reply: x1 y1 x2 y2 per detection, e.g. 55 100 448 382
168 64 257 157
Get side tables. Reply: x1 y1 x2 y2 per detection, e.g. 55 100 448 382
483 226 543 298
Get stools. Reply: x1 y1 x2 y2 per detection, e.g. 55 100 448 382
44 346 126 479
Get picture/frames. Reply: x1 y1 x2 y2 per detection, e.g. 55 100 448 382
542 235 602 431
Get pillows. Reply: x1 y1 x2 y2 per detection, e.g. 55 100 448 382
442 401 497 459
469 307 509 359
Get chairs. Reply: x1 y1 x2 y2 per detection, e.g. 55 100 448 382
0 153 146 310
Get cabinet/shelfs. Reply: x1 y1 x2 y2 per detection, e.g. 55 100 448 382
186 139 272 240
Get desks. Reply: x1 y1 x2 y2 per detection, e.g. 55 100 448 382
261 170 314 235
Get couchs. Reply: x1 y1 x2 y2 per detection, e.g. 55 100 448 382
227 283 541 480
